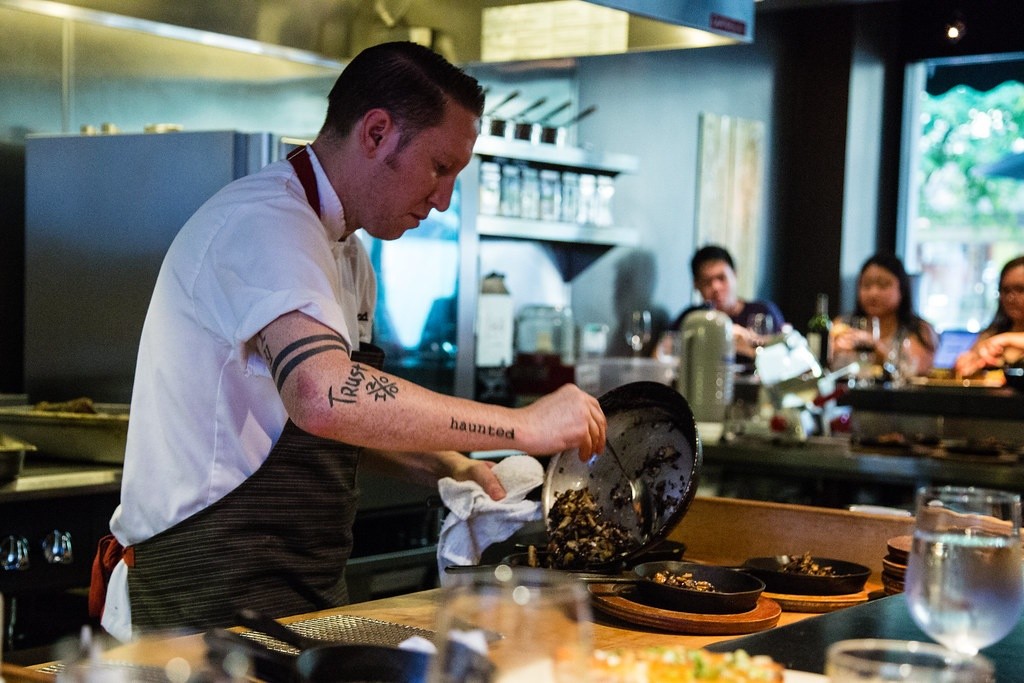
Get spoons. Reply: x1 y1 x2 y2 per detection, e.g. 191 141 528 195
606 437 651 527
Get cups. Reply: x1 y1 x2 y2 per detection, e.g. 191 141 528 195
747 311 775 346
825 638 995 683
903 485 1023 655
425 566 592 683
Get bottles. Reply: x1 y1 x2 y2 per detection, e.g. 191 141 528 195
806 294 833 372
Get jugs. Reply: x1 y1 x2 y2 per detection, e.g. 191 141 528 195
677 297 736 423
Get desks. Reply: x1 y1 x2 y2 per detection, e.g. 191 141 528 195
0 491 924 681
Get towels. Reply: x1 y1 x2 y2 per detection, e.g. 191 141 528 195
435 453 545 586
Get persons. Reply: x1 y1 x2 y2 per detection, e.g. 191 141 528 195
97 40 605 644
952 256 1024 382
822 250 938 381
644 245 786 361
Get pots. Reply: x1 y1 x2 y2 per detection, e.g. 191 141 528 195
568 560 767 614
721 556 871 596
235 608 489 683
527 381 703 571
445 540 687 575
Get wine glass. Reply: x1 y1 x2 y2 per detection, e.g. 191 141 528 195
851 316 881 387
623 308 652 358
